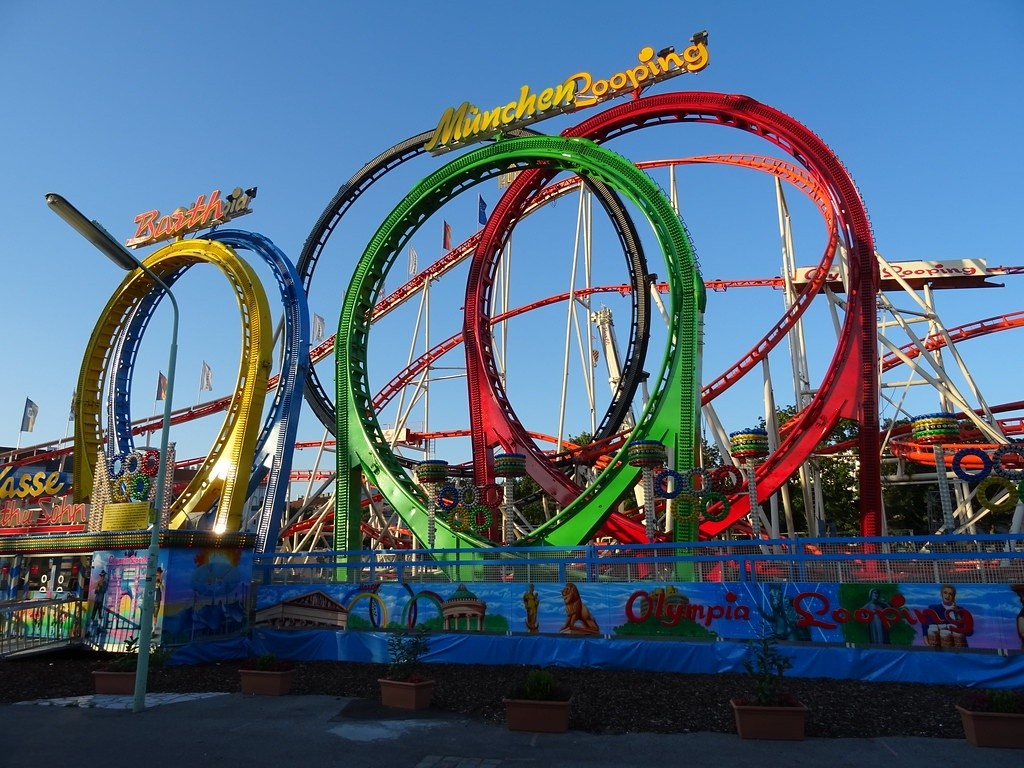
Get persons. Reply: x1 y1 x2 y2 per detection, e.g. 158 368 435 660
524 584 540 633
756 583 811 641
922 585 974 652
862 588 892 644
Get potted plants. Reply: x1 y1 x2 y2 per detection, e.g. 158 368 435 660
501 668 574 733
238 653 296 696
954 691 1024 748
729 656 808 741
377 626 435 710
93 632 189 695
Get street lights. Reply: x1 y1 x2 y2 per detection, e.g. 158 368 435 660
45 192 179 712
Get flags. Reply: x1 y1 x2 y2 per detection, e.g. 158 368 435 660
409 246 417 275
68 392 77 421
442 221 451 251
311 314 325 342
20 399 39 432
200 362 213 391
155 372 167 400
376 280 386 296
479 194 488 225
